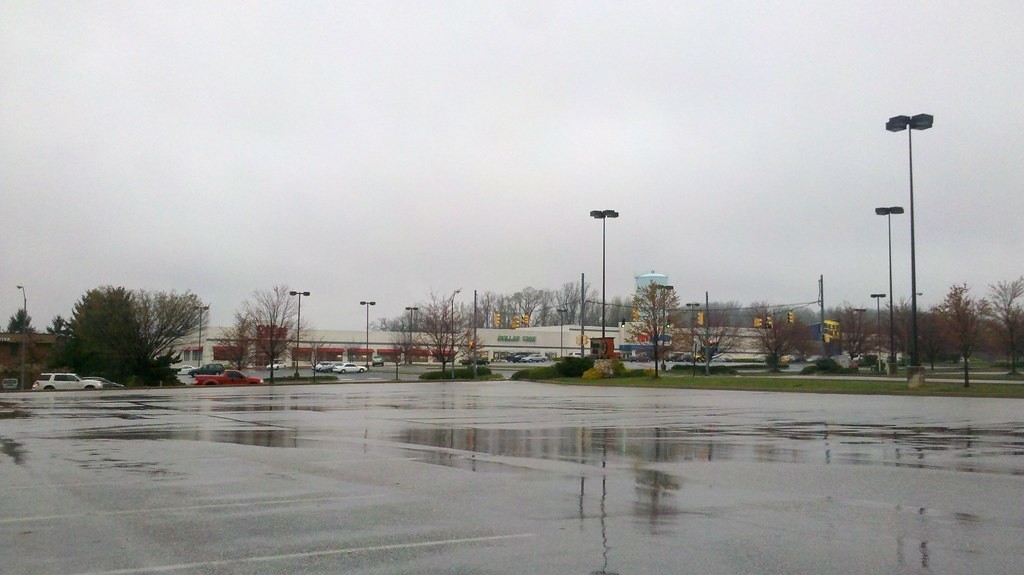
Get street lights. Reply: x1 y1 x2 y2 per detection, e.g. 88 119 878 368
16 283 27 391
289 291 311 379
886 113 935 391
875 206 905 377
685 303 699 363
655 284 674 370
871 293 886 372
194 305 209 365
588 207 623 376
450 290 462 379
405 306 419 364
360 301 376 369
556 308 567 359
855 308 867 353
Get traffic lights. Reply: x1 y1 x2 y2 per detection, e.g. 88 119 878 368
787 312 793 324
524 316 528 324
495 313 500 324
765 316 773 330
753 318 763 328
522 316 525 324
513 316 519 328
823 334 830 343
621 318 625 328
704 339 713 346
697 312 704 326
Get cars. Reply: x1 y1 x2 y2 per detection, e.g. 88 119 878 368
629 351 734 364
569 352 582 359
462 357 490 365
309 361 342 374
175 365 198 375
333 363 368 374
2 378 18 391
82 376 125 389
776 352 878 373
267 358 287 371
520 354 548 363
506 353 528 363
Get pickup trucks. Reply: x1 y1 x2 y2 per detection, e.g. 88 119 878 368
194 369 265 386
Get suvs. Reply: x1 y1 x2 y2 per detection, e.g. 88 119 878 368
188 363 226 377
372 356 385 367
31 372 103 391
